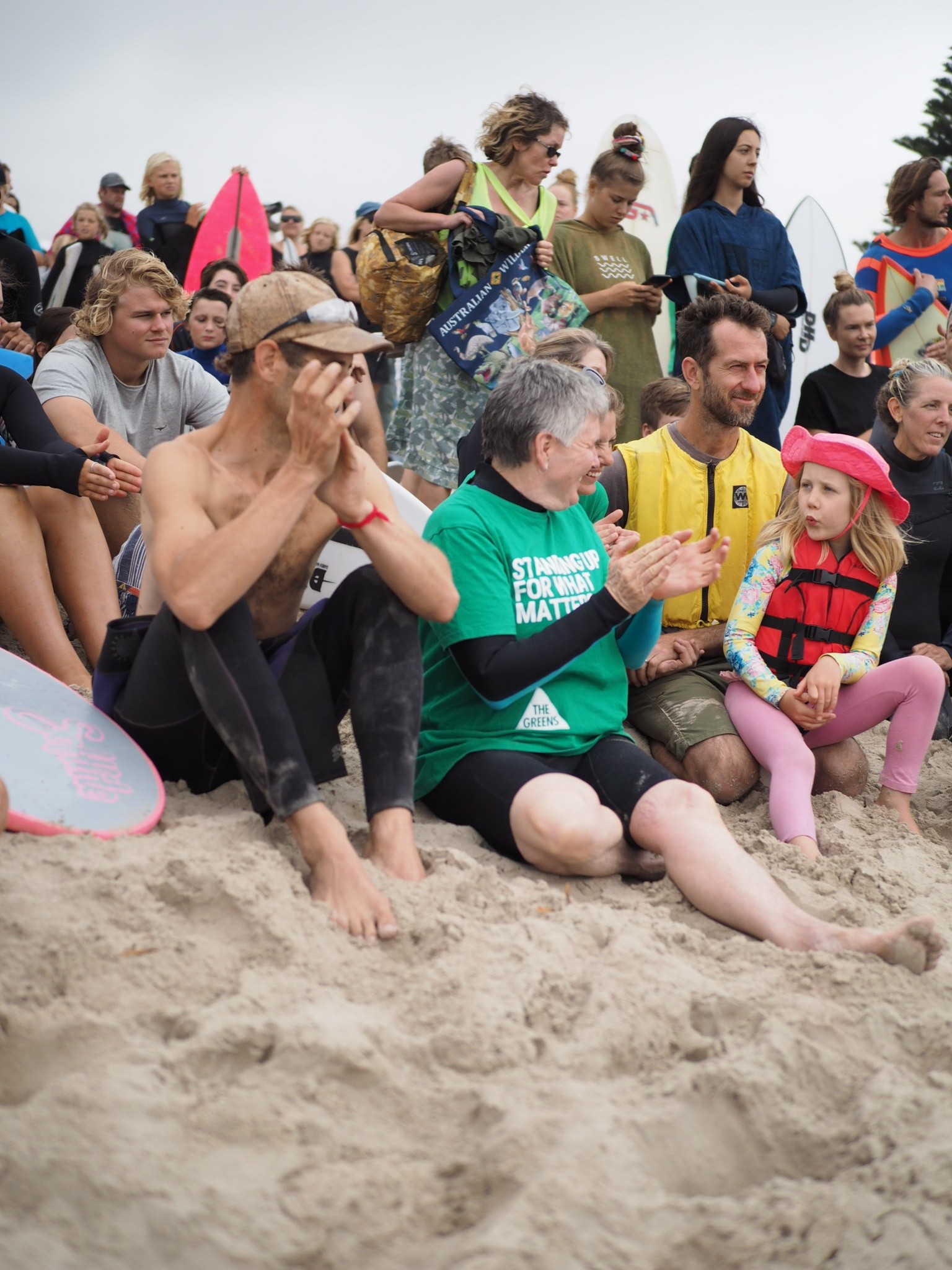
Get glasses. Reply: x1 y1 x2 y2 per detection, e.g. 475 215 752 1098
281 215 302 223
535 138 561 159
261 298 360 340
583 366 606 388
368 215 374 223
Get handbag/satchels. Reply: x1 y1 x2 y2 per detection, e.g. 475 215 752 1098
428 205 590 391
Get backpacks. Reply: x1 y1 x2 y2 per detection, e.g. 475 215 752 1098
356 155 478 343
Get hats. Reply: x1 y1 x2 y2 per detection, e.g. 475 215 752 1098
356 201 383 217
226 270 396 356
781 425 910 525
100 172 130 190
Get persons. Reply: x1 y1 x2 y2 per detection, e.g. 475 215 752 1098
723 425 946 859
114 269 459 941
417 358 951 973
1 92 951 804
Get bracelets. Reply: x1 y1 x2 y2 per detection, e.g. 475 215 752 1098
336 502 389 530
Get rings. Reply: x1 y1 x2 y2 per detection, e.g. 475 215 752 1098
91 462 98 473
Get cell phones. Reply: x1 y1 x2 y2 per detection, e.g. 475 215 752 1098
693 273 726 286
641 275 671 288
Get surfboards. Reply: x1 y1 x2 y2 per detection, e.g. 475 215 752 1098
0 647 166 838
575 115 670 380
183 172 273 292
777 195 849 450
875 254 949 369
